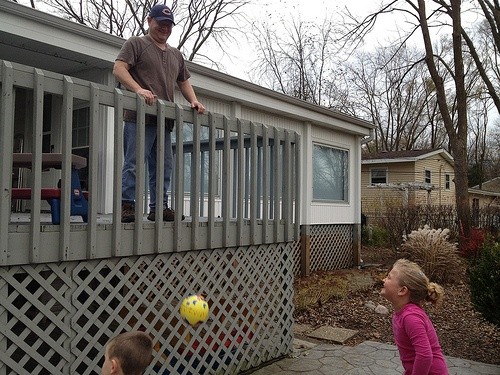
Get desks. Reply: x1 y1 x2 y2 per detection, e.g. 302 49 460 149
14 153 88 223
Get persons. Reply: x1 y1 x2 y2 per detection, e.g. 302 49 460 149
102 331 153 375
380 259 449 375
113 5 206 223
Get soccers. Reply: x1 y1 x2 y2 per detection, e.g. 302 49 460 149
179 295 208 327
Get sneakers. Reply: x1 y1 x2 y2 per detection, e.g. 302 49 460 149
121 209 135 223
148 207 185 222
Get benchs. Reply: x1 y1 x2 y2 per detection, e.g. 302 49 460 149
11 188 89 224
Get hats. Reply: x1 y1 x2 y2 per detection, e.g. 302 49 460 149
150 5 175 26
